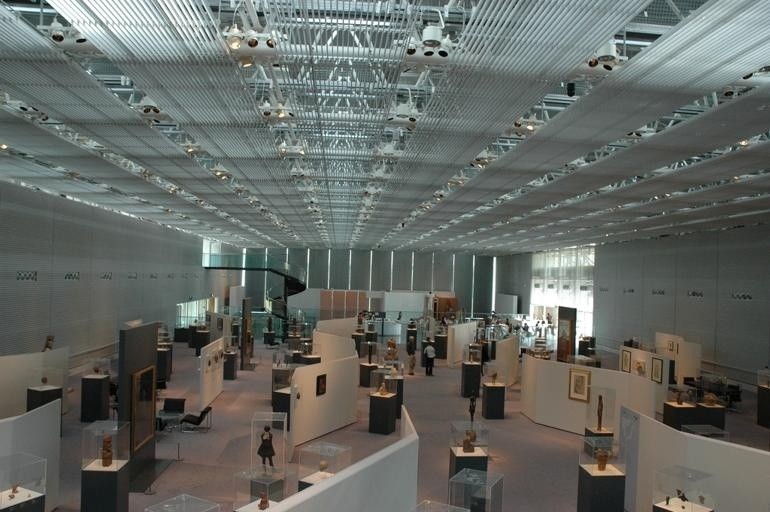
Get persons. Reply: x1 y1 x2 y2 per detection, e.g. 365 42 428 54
257 424 280 472
424 342 437 376
358 313 364 325
407 336 417 375
438 316 448 335
469 390 477 422
490 313 556 337
397 311 403 320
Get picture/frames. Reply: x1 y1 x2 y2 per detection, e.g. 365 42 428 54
206 314 210 322
621 350 631 373
317 374 326 396
651 357 663 383
205 347 223 370
668 340 673 351
217 318 223 329
568 368 591 403
132 366 154 452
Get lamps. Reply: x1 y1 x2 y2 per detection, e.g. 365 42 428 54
0 0 331 249
349 0 770 254
